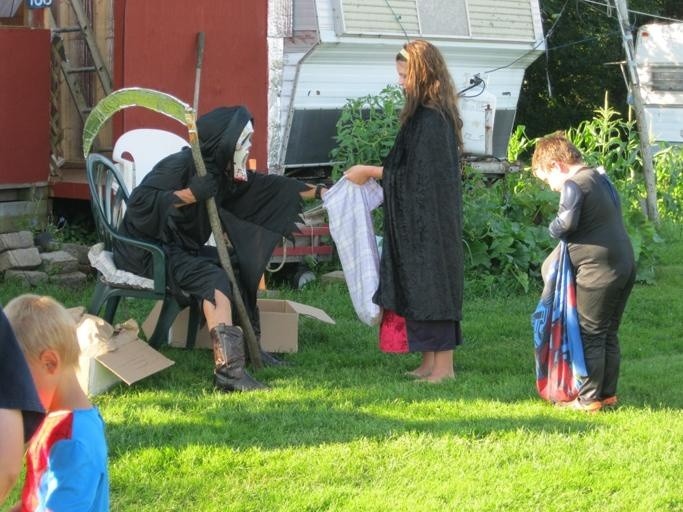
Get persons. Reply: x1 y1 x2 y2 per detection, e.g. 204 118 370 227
113 103 334 398
0 308 46 512
3 293 110 512
341 37 465 387
529 136 638 416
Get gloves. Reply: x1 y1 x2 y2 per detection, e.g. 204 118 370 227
189 172 217 201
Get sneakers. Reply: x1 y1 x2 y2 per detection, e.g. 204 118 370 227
554 396 617 414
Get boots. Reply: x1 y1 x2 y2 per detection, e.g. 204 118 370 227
211 322 266 392
243 332 293 366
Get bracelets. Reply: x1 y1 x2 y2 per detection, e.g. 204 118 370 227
314 180 327 200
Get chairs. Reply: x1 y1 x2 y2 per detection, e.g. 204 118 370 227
84 126 222 351
